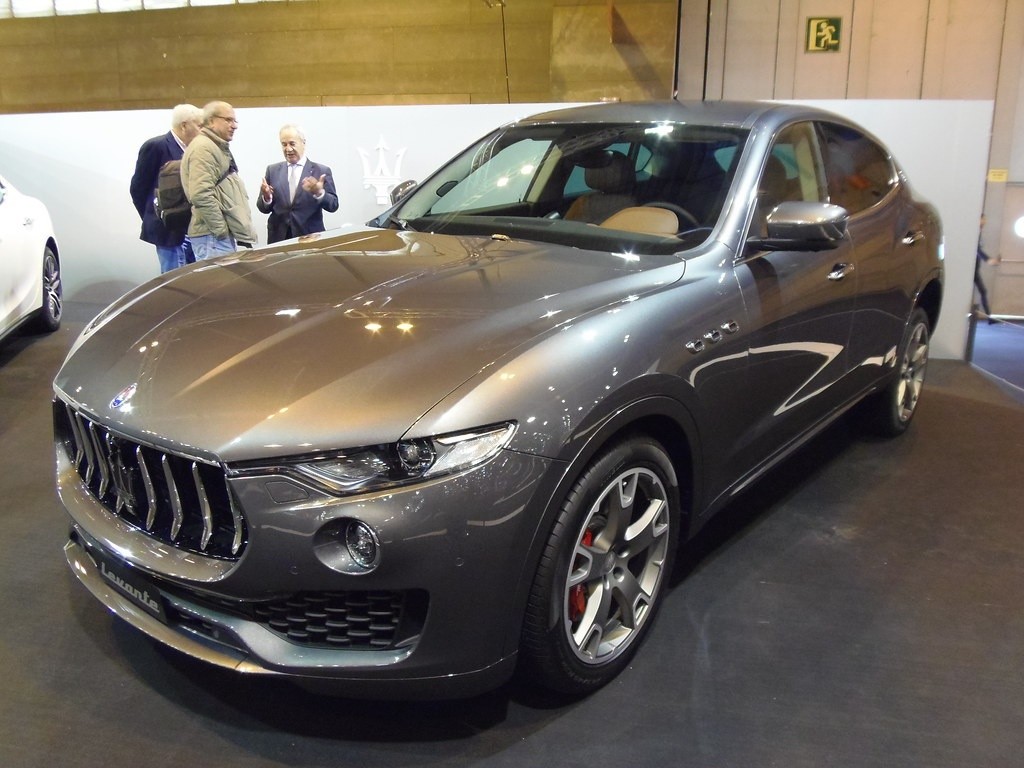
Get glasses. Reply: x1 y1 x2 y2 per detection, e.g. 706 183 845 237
212 114 237 123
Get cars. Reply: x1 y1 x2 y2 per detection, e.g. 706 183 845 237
0 173 62 344
50 95 944 702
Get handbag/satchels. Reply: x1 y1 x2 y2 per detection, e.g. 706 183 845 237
154 159 191 229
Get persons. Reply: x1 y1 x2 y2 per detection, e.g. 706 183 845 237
129 100 258 278
256 124 340 247
974 214 1004 325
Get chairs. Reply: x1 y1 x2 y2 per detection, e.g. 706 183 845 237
753 156 790 237
562 150 639 227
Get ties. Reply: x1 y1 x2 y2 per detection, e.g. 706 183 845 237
289 163 298 202
